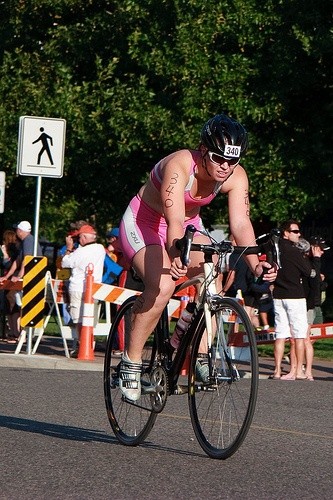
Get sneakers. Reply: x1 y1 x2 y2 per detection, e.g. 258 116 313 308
196 352 210 383
119 350 143 401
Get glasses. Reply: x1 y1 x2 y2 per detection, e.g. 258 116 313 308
289 230 300 234
205 147 241 167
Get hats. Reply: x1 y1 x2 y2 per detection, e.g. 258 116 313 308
12 221 31 233
258 254 266 261
106 228 120 237
71 224 97 237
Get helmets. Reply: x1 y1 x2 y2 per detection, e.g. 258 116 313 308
200 114 250 159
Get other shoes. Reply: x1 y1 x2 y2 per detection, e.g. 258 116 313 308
306 376 314 381
280 373 295 381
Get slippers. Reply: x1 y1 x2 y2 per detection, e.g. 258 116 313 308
268 375 280 379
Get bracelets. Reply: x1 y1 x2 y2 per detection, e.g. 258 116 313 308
67 249 71 252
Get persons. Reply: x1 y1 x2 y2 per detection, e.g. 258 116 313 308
206 226 272 334
315 234 333 324
0 229 25 345
115 116 280 401
58 219 129 357
281 237 323 381
270 217 318 382
0 219 43 283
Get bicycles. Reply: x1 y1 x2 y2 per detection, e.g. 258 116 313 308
105 222 284 460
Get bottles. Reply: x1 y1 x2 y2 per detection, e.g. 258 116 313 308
168 301 197 349
